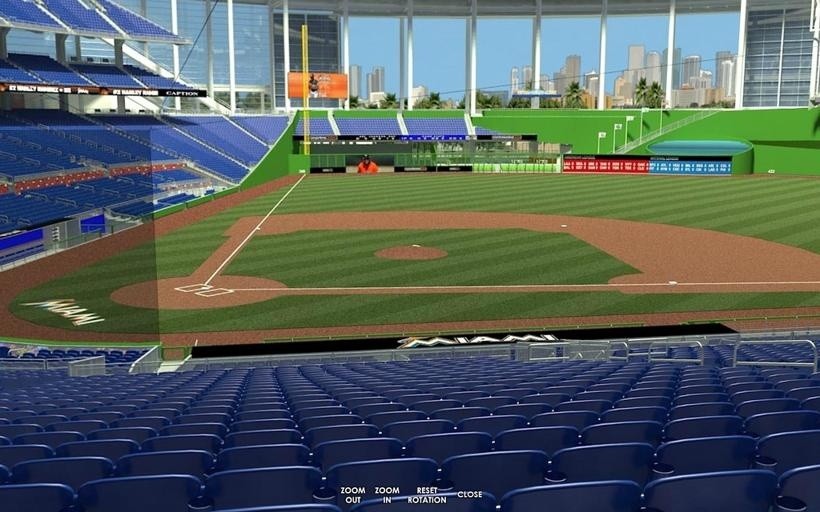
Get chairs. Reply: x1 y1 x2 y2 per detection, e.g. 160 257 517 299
294 115 508 141
